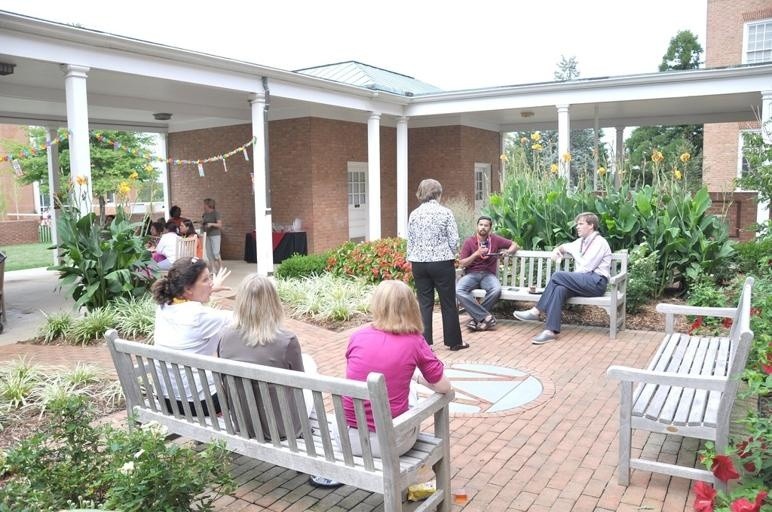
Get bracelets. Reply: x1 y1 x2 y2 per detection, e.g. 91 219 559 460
417 374 420 384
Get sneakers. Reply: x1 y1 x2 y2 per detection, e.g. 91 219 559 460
450 343 469 351
467 320 477 330
478 316 497 331
532 330 556 344
513 310 540 321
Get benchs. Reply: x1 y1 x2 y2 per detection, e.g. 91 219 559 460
453 248 632 340
103 324 452 512
608 275 757 495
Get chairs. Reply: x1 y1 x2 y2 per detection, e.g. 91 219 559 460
155 234 198 280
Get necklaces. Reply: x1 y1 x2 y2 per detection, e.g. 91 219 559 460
172 297 190 303
579 232 599 257
476 234 492 261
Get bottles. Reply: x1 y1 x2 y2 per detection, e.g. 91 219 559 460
481 242 486 257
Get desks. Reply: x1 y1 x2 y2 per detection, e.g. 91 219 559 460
245 230 308 264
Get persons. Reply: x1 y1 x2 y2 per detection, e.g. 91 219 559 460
149 256 237 416
513 211 612 345
338 278 451 459
406 177 469 352
454 215 517 331
98 205 199 276
202 197 224 270
212 273 320 442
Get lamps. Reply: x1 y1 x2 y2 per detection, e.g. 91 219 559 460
1 61 17 75
152 111 172 122
